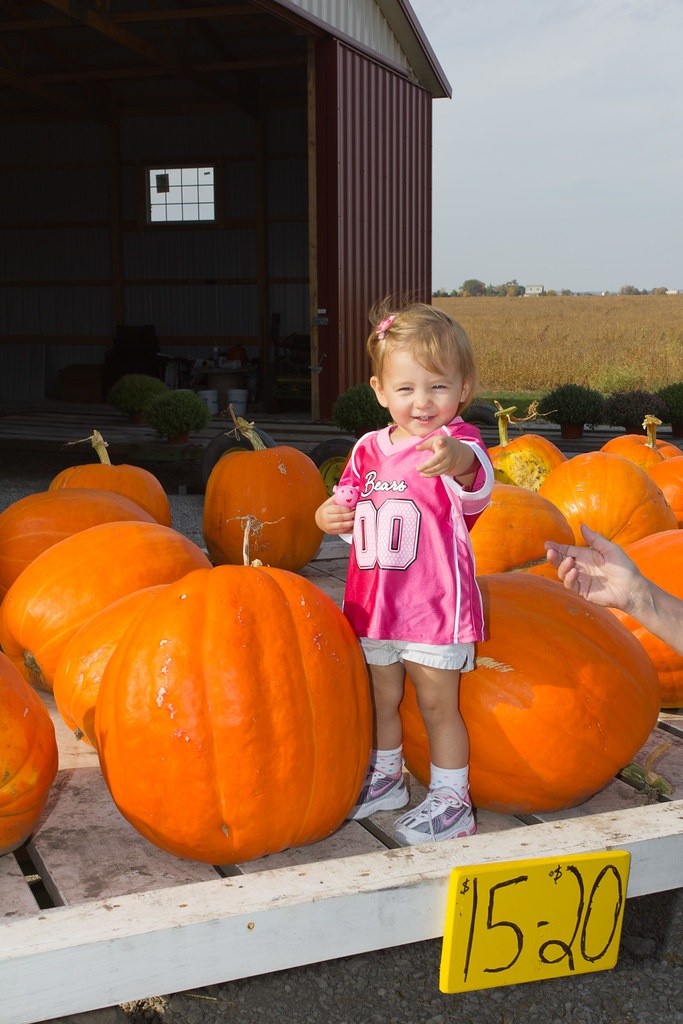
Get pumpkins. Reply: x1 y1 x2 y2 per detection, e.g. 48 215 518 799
0 400 683 864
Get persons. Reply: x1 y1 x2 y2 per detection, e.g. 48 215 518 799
317 299 496 844
541 521 682 663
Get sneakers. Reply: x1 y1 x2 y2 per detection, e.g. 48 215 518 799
392 783 476 846
345 765 409 820
